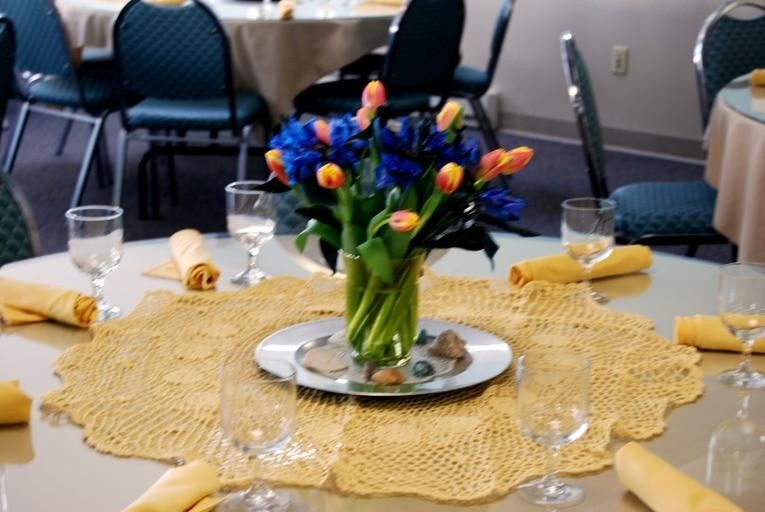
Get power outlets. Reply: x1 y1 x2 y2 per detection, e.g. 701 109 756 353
610 45 628 75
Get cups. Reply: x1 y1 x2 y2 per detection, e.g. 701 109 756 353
706 425 765 512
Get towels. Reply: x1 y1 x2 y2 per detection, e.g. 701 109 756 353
614 442 745 512
675 315 765 354
169 228 222 290
120 459 236 512
0 276 100 330
508 244 651 288
0 379 33 424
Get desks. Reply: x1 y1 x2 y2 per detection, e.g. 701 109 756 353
57 4 409 130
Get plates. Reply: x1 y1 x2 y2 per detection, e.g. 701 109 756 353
257 314 511 395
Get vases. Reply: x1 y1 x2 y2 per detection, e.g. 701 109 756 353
339 247 425 368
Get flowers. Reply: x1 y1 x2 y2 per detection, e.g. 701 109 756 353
255 81 535 359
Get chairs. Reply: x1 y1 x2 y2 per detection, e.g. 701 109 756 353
290 1 467 146
112 1 272 235
1 2 163 215
429 1 517 210
560 28 742 264
690 1 764 138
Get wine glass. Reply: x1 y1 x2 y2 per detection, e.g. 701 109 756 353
224 180 275 286
712 259 765 391
515 351 589 505
215 357 302 510
65 206 125 324
559 197 614 303
